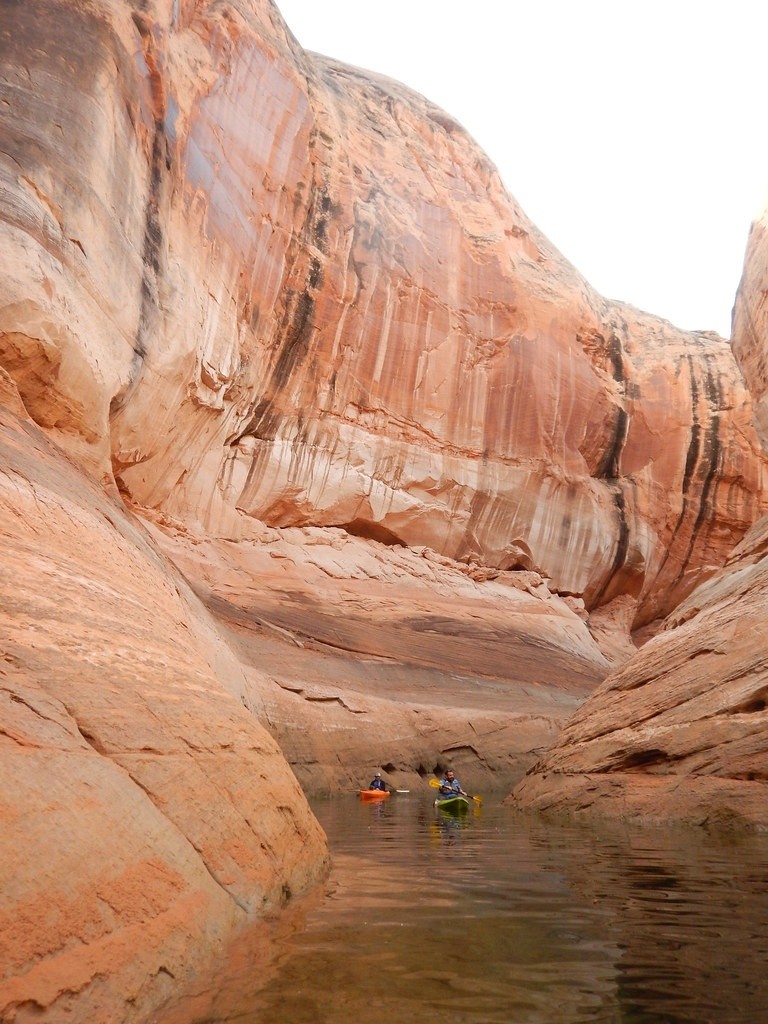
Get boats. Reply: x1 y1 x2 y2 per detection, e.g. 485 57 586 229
360 789 390 799
436 797 469 811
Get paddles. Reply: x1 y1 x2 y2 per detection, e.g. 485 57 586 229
429 778 482 807
348 789 410 793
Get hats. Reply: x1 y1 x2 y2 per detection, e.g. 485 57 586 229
374 773 381 777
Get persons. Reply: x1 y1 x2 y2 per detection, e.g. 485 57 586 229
437 769 467 801
370 772 385 791
